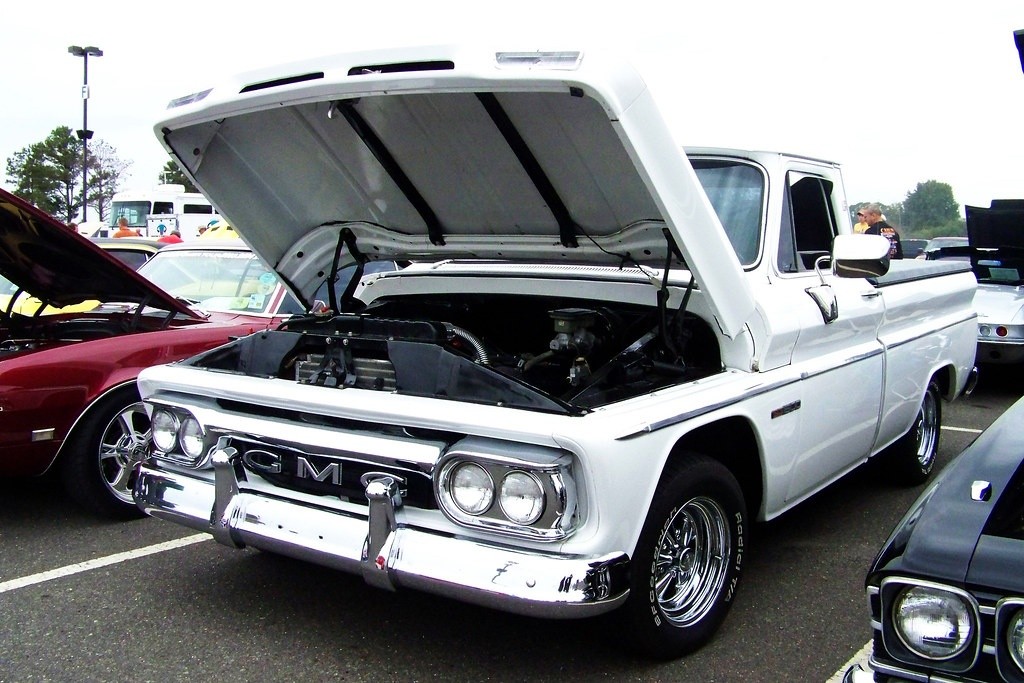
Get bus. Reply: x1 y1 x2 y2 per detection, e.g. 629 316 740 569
106 184 221 237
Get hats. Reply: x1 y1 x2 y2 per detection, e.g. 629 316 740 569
196 225 206 231
857 209 865 216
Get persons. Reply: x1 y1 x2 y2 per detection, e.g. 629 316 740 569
197 225 208 234
112 217 143 238
158 230 185 244
68 223 79 232
854 205 903 260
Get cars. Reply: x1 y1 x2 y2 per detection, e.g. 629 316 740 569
865 394 1024 683
896 198 1024 360
133 48 980 663
0 190 403 521
197 220 239 237
0 237 263 337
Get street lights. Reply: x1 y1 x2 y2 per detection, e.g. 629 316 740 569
67 44 104 227
